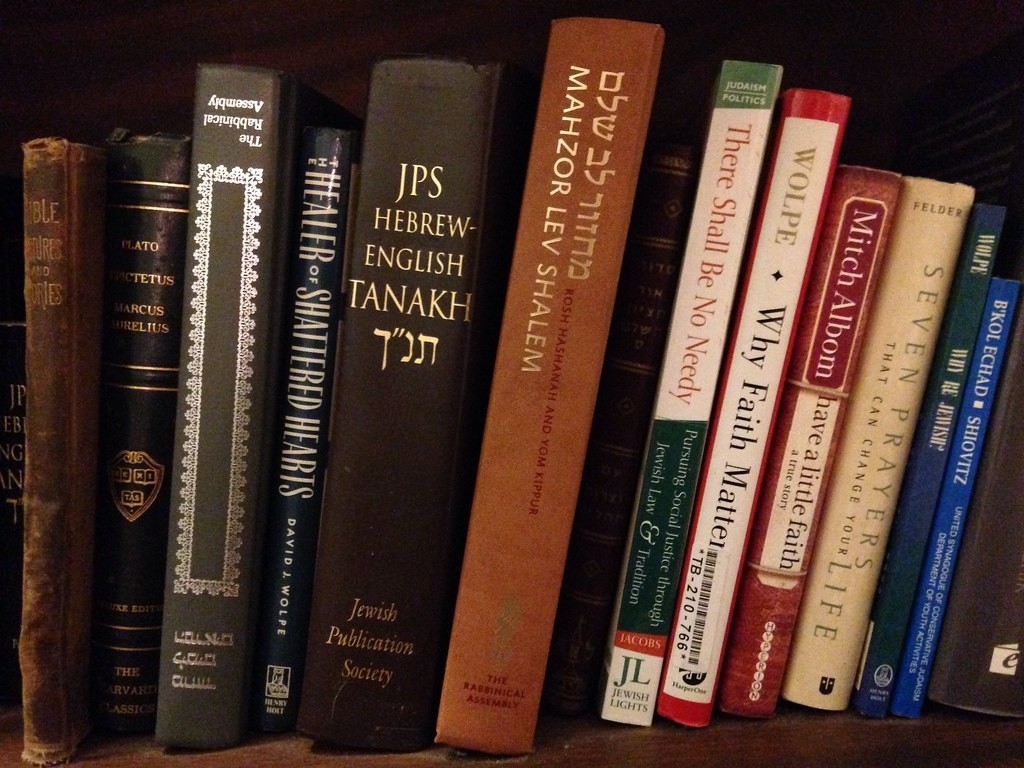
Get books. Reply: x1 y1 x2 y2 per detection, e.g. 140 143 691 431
0 16 1024 768
